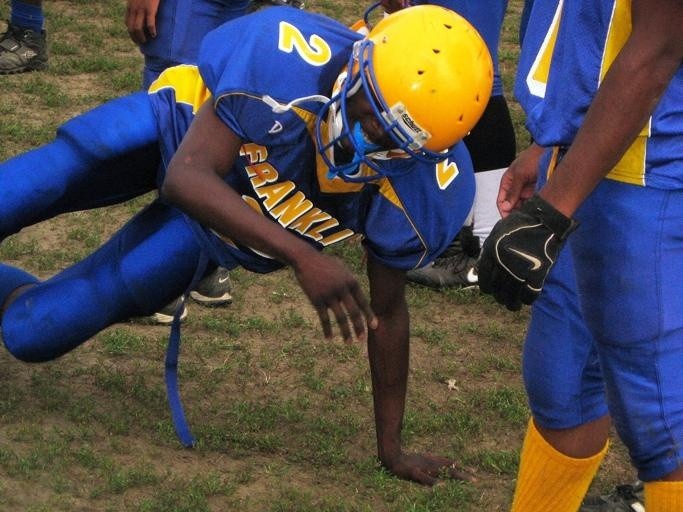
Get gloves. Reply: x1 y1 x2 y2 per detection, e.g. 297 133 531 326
477 192 580 312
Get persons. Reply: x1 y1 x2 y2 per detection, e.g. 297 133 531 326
125 0 301 329
0 5 495 487
380 0 518 294
472 0 683 511
0 0 48 76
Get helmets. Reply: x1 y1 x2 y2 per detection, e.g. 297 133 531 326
316 3 493 184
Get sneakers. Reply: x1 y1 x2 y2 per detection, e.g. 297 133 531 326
0 22 49 74
134 263 232 325
579 478 645 512
407 245 511 292
